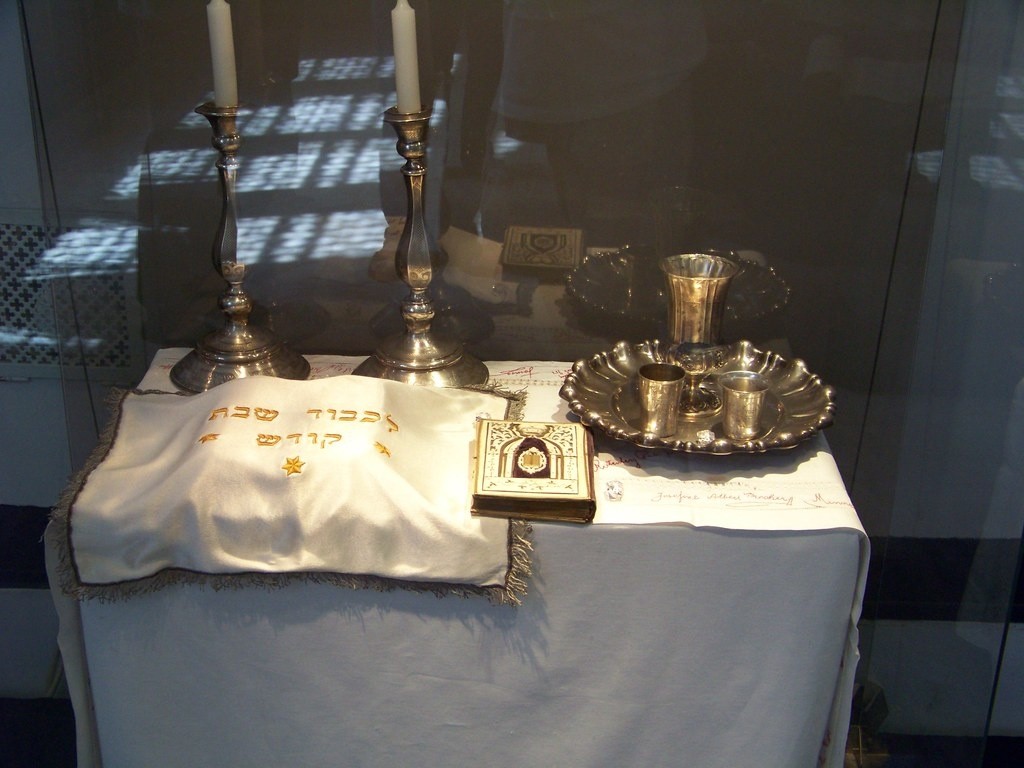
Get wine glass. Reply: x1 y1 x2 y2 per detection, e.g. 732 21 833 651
659 253 741 416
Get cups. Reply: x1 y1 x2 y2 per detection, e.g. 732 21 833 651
638 363 685 437
717 371 772 441
602 251 637 299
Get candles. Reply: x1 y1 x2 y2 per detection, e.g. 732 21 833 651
205 0 239 110
391 0 421 115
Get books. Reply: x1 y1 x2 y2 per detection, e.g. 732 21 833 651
502 226 583 284
470 419 596 523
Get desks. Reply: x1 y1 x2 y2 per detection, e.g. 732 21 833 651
44 348 869 767
